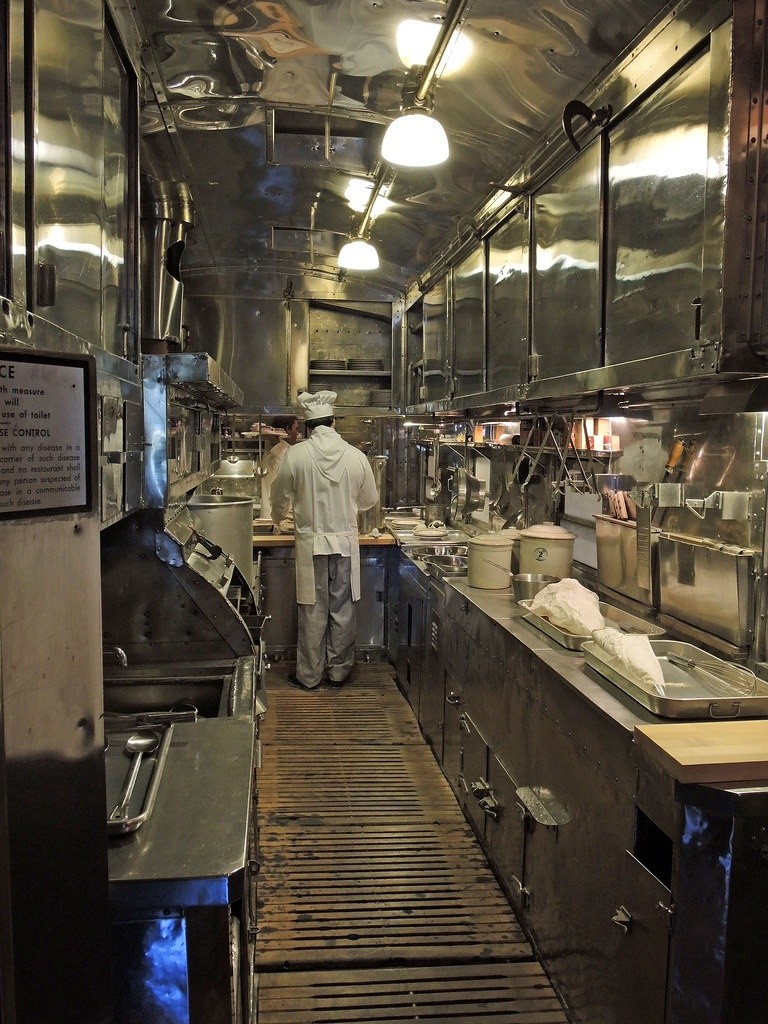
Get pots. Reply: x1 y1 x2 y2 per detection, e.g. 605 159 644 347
449 468 486 520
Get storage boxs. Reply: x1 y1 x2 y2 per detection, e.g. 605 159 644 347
574 418 620 451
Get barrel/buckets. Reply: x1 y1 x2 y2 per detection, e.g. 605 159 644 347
176 494 256 589
357 454 388 534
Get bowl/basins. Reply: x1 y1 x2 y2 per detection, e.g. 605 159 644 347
420 555 469 572
411 544 468 560
479 558 560 600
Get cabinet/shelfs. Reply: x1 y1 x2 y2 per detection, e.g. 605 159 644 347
309 369 391 418
399 0 768 416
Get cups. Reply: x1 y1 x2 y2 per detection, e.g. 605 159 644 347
468 529 513 589
497 526 523 574
518 520 578 579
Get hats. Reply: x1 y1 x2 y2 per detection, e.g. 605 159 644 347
297 390 337 421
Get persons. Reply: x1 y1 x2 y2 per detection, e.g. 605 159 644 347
270 390 378 692
260 415 299 521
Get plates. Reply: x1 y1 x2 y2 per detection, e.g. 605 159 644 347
392 518 426 525
413 530 449 539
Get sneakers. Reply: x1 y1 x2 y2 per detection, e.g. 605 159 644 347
330 672 350 689
287 672 318 691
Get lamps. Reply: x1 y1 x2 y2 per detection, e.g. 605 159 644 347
337 228 380 271
381 96 450 167
